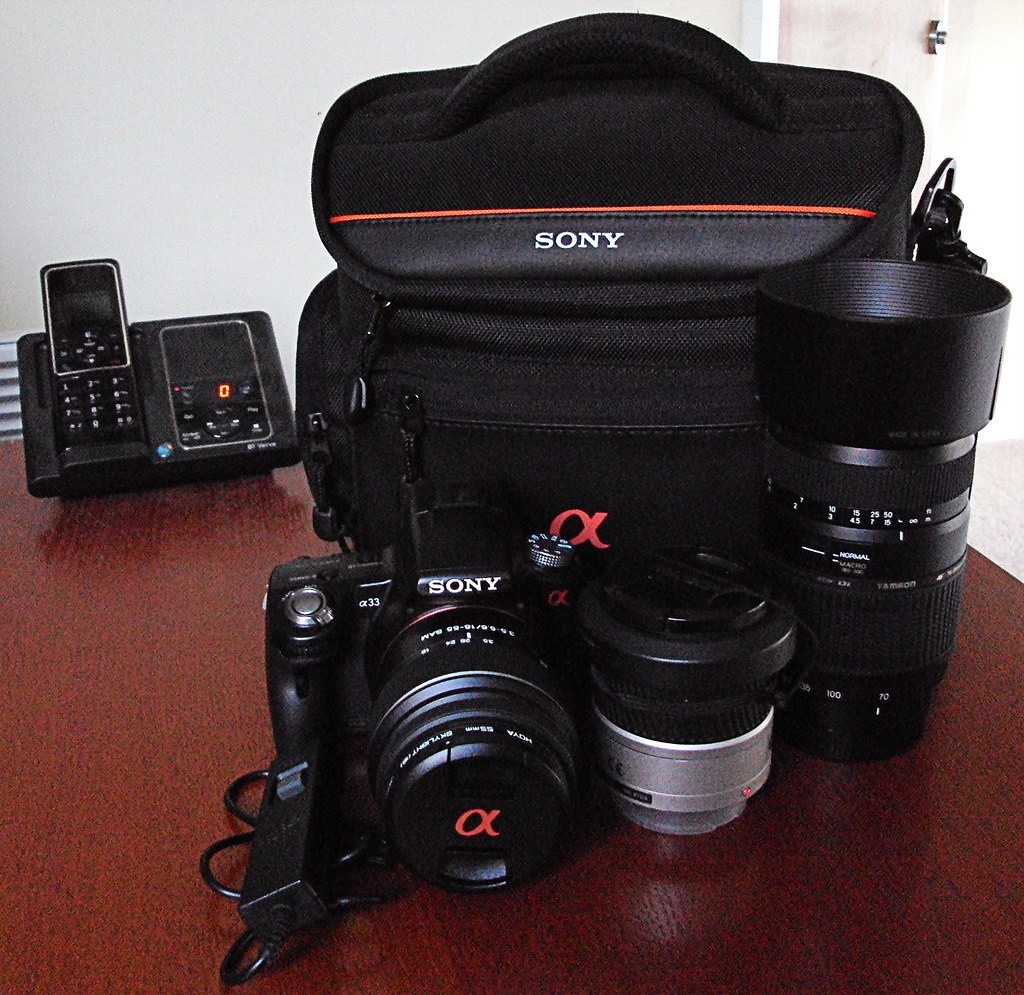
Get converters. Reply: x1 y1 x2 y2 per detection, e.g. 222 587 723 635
239 732 340 940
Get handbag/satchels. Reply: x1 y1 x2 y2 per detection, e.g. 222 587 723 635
293 12 926 587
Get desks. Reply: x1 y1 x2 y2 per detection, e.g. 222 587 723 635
0 433 1024 995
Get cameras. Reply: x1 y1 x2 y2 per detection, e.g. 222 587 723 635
248 498 804 901
